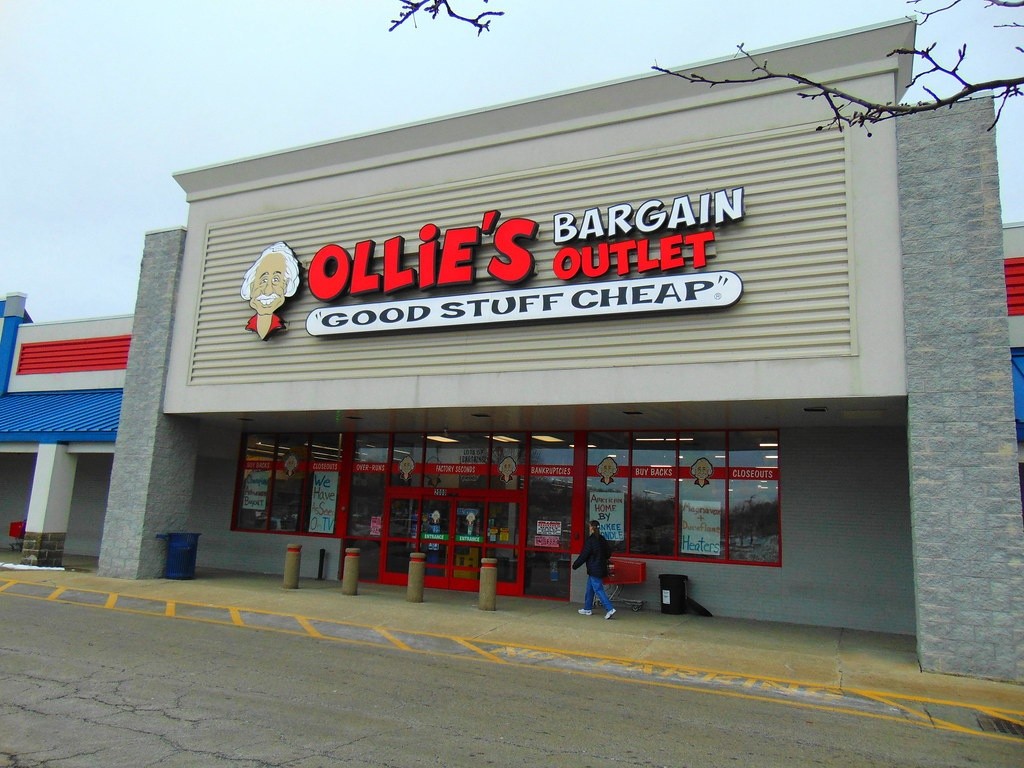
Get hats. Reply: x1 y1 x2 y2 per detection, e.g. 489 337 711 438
586 519 601 529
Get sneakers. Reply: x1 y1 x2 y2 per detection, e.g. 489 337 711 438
604 608 616 620
577 609 592 615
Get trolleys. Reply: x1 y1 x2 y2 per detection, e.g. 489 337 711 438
592 557 647 612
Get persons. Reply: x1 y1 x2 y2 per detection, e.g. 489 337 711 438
572 520 616 620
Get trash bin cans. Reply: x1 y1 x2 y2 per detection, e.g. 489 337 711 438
155 532 202 580
658 574 689 615
